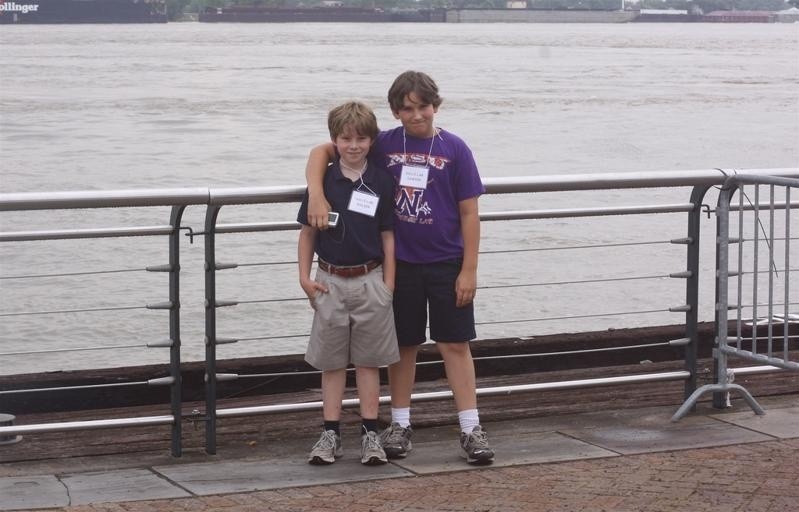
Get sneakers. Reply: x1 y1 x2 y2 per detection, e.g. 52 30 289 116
380 419 414 459
456 424 496 466
307 427 345 466
359 424 388 466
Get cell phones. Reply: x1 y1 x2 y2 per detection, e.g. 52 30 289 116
328 211 340 228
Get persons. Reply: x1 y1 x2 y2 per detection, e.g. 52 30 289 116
296 101 402 464
305 70 495 464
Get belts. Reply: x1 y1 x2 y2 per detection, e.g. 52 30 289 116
315 254 384 280
